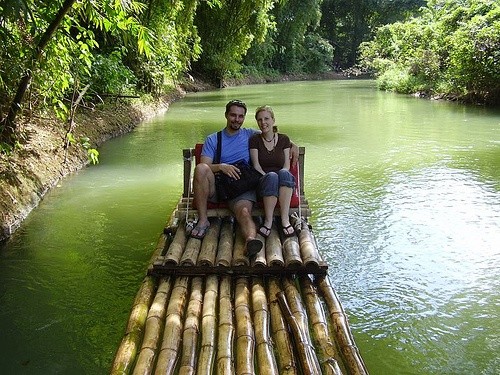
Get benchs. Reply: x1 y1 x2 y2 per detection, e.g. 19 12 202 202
175 144 311 218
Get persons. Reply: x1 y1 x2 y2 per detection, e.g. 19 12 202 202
248 104 295 238
191 99 299 256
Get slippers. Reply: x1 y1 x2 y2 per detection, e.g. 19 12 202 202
282 224 295 237
246 239 263 257
191 223 210 238
257 225 271 238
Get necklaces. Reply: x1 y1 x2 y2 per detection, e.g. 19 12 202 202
262 131 276 144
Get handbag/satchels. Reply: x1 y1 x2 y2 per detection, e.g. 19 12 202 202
223 158 263 199
